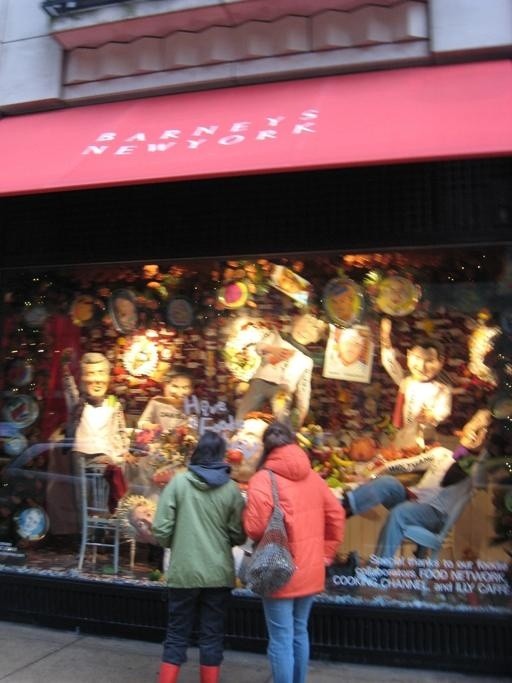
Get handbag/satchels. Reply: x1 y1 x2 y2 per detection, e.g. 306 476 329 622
243 507 295 596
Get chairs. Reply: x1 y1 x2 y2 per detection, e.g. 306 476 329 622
72 455 146 576
396 485 473 597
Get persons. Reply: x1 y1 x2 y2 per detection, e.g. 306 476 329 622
329 284 355 323
330 326 369 377
338 410 503 592
151 432 249 683
233 309 329 429
136 365 195 433
61 347 129 531
242 422 346 683
379 318 453 429
